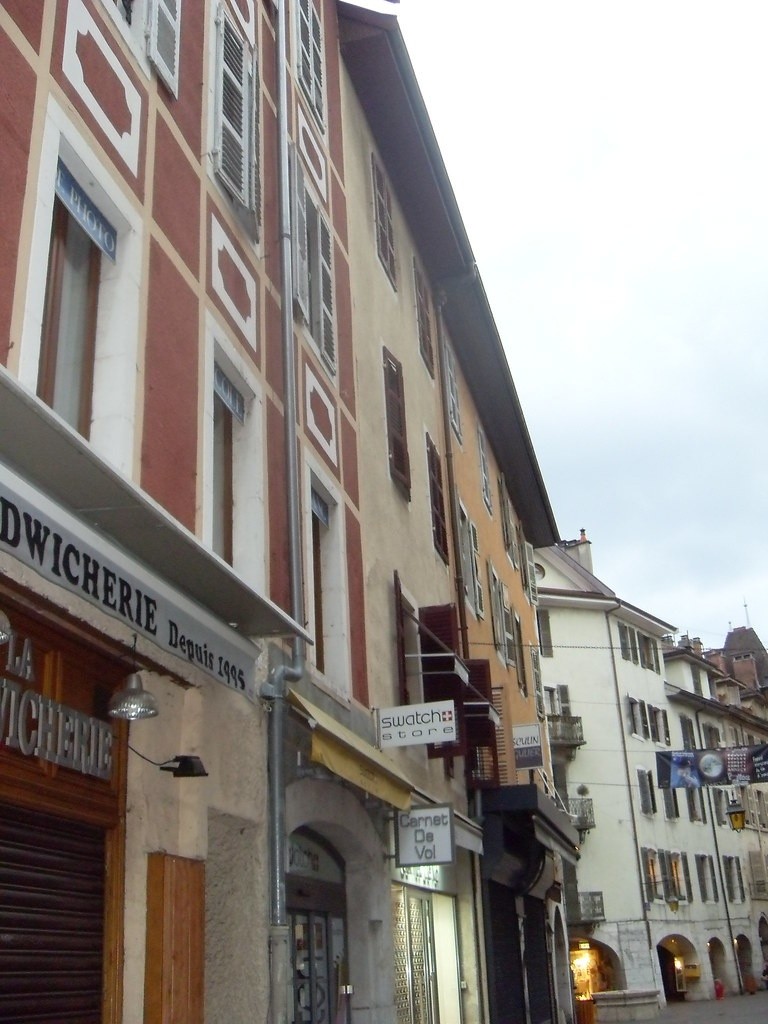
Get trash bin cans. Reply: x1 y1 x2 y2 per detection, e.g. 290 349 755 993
575 999 596 1024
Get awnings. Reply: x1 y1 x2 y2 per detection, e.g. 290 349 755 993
409 790 485 856
286 688 415 816
2 374 311 704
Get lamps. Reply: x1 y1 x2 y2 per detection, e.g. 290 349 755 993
125 742 211 779
339 983 354 998
718 787 746 833
639 877 679 914
104 648 160 721
579 939 591 951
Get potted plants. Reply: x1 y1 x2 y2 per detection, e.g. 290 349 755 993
574 990 597 1024
